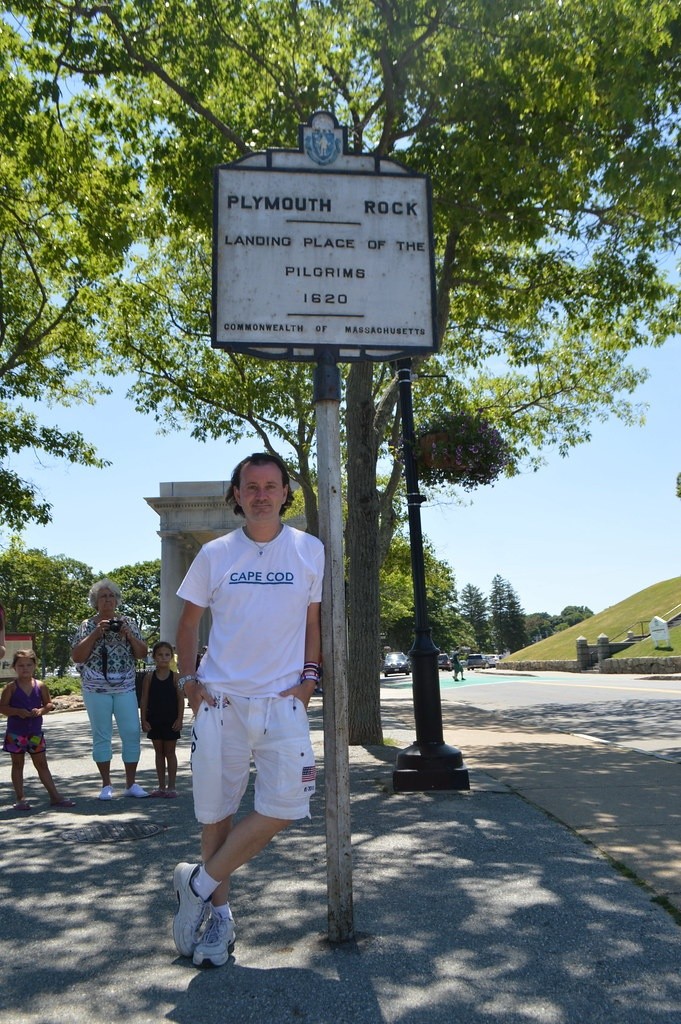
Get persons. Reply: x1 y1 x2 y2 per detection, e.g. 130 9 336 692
71 579 149 800
0 649 75 811
140 642 185 798
452 646 466 681
171 453 324 968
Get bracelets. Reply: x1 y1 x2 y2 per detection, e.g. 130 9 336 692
301 662 320 686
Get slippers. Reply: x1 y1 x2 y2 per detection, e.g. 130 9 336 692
14 802 31 810
51 797 76 807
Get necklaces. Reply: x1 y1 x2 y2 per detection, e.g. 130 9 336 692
245 525 282 557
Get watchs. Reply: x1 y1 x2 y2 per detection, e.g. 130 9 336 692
177 674 196 690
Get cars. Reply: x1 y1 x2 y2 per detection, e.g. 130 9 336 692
485 655 503 668
383 652 411 677
466 654 486 671
438 653 453 671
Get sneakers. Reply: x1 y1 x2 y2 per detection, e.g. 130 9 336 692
193 908 235 967
125 783 150 798
173 862 213 957
99 786 113 800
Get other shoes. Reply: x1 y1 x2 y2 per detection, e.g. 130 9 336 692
152 789 164 796
164 791 176 798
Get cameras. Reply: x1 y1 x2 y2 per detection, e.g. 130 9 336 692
107 619 123 633
